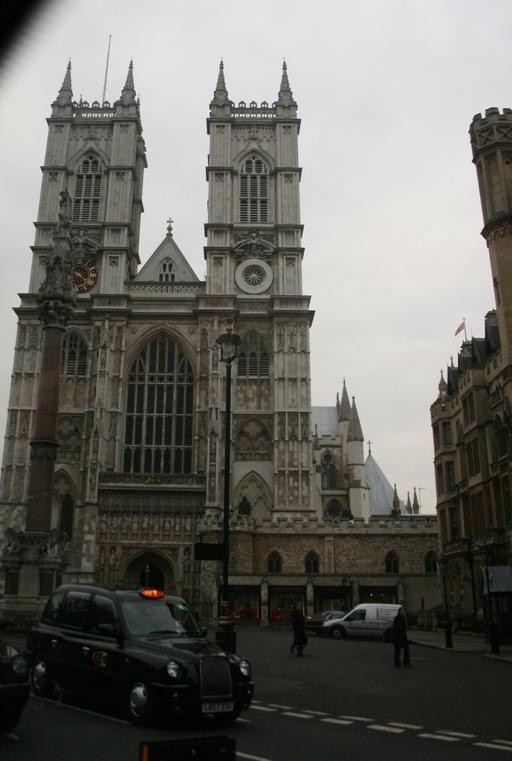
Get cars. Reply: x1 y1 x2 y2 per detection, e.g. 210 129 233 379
305 609 345 635
30 584 254 730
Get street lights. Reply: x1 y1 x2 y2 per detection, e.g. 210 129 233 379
216 319 240 653
476 537 500 654
437 557 453 649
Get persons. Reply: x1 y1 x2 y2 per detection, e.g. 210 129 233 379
289 599 306 656
288 604 294 615
272 607 281 619
228 606 240 625
393 607 409 667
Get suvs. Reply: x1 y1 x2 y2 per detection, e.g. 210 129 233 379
1 640 52 741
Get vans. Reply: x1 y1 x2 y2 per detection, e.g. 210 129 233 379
321 603 404 639
